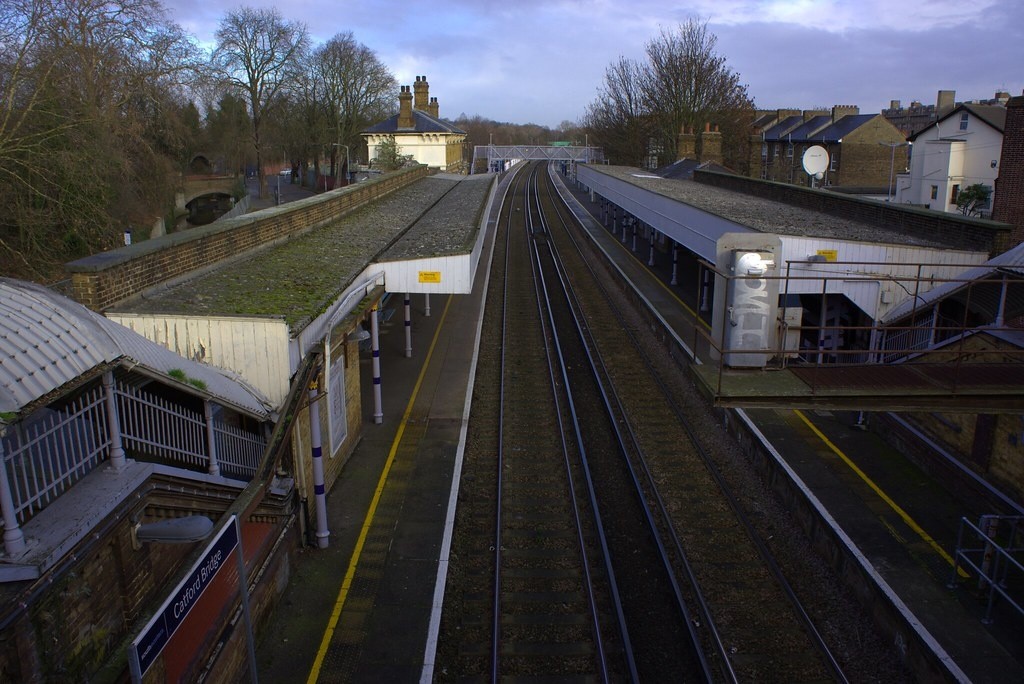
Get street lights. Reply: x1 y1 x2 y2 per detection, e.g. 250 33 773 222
331 143 351 185
878 141 908 200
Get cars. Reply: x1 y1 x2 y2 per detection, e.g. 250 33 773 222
279 168 292 176
